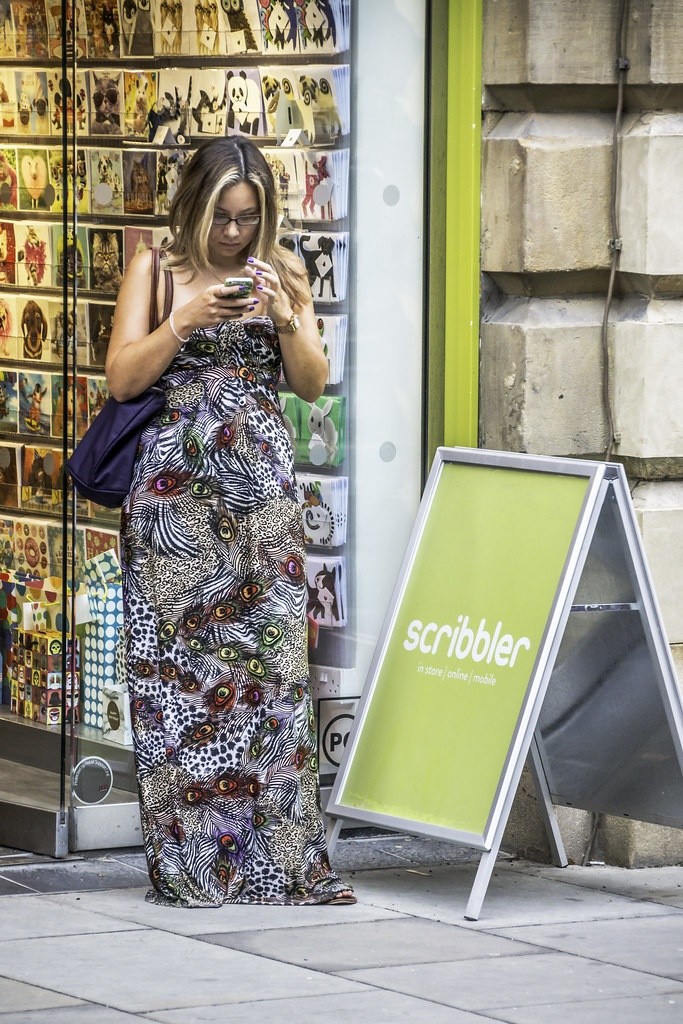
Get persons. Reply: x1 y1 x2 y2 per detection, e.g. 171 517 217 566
98 137 356 905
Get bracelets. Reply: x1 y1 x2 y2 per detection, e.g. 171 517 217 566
170 311 190 352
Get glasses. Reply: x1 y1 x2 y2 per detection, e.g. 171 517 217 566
210 214 261 225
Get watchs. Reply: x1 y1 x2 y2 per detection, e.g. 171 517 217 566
274 311 301 335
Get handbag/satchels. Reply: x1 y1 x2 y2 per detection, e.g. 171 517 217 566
0 568 136 745
64 247 174 508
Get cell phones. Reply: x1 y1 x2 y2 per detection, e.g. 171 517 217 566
224 277 253 298
